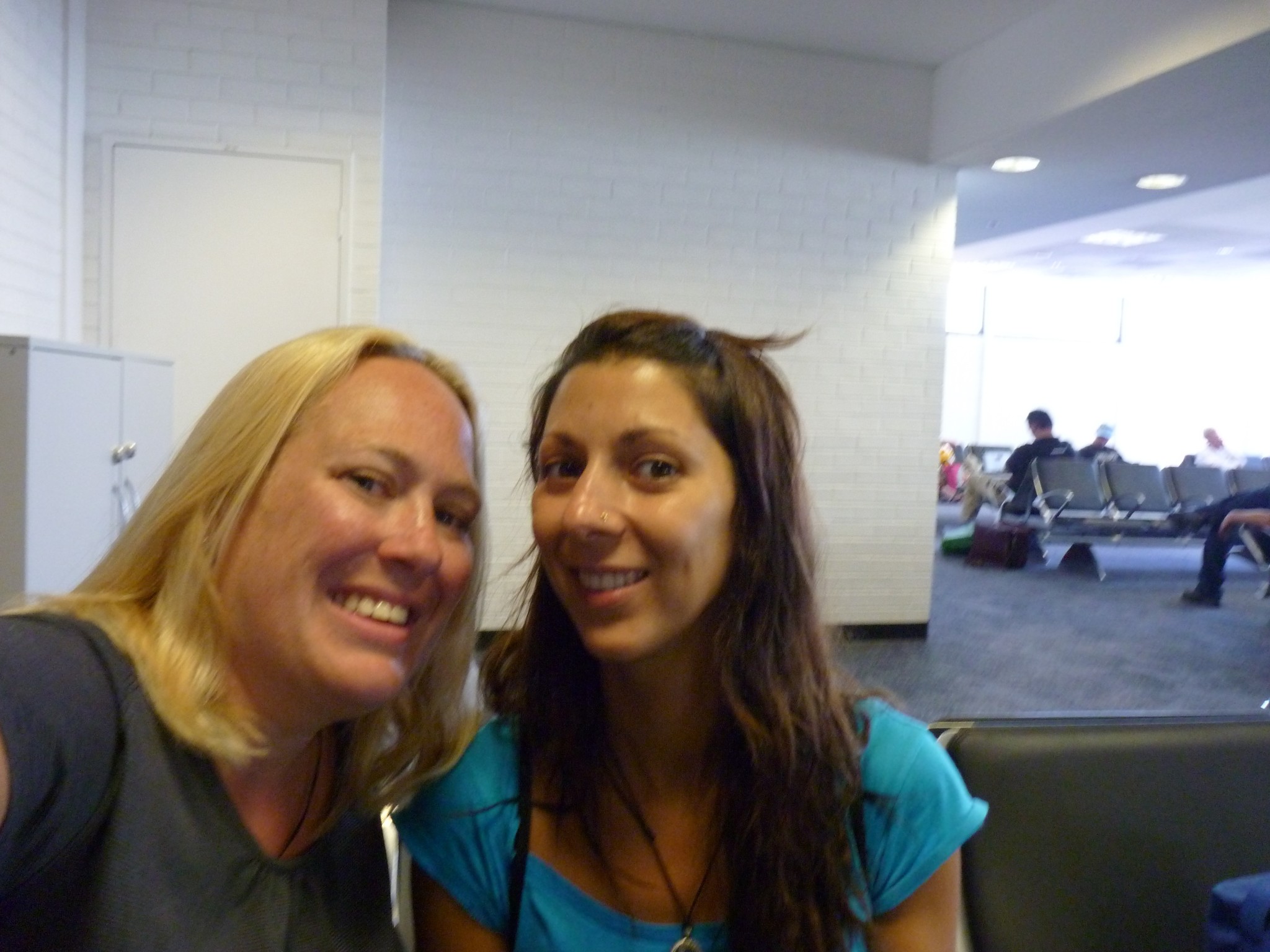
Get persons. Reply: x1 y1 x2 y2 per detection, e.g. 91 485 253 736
993 408 1269 609
0 322 491 952
394 314 989 952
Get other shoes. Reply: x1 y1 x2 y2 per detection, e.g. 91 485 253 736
1182 588 1221 607
1169 511 1204 530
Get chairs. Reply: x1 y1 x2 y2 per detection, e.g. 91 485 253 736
931 712 1270 952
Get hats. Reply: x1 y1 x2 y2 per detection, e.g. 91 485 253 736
1095 425 1114 439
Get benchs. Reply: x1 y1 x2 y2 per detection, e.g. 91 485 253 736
939 445 1270 597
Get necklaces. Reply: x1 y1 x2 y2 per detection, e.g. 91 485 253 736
596 715 753 952
